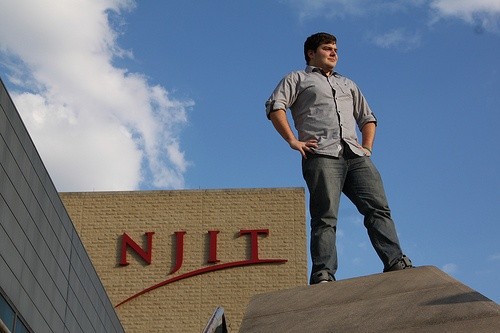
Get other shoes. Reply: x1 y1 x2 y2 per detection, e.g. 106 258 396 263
383 254 417 272
310 271 334 284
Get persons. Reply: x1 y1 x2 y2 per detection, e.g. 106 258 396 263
265 32 417 286
